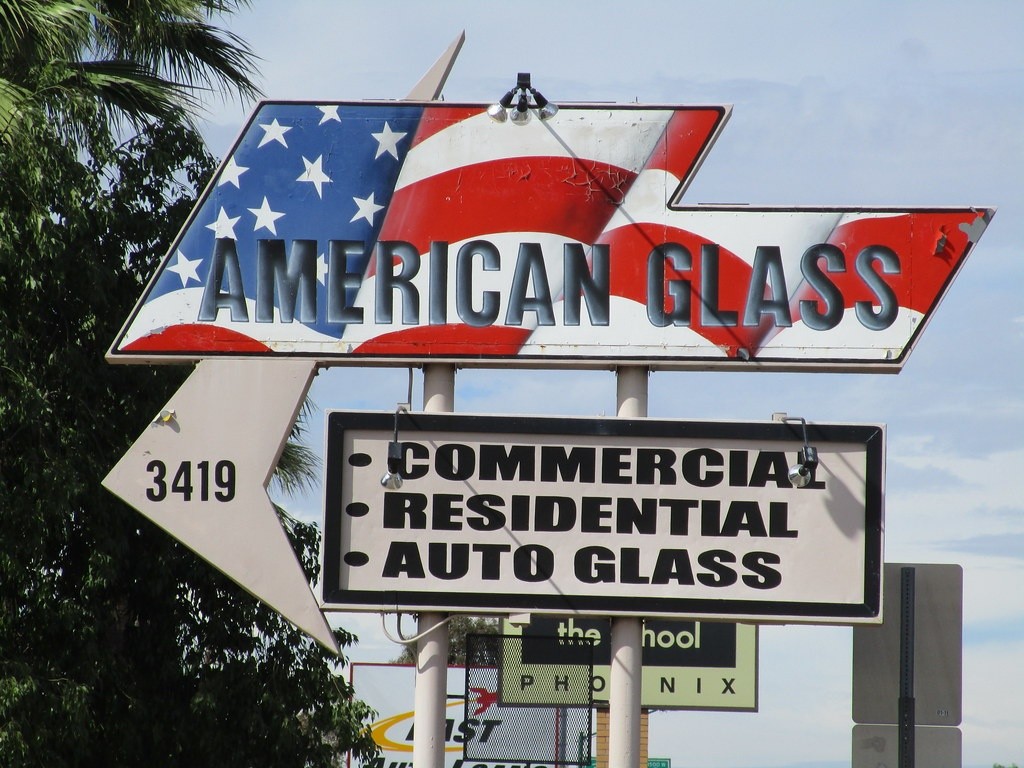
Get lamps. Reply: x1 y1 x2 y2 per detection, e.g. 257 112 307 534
773 412 819 487
489 74 559 123
380 401 409 490
